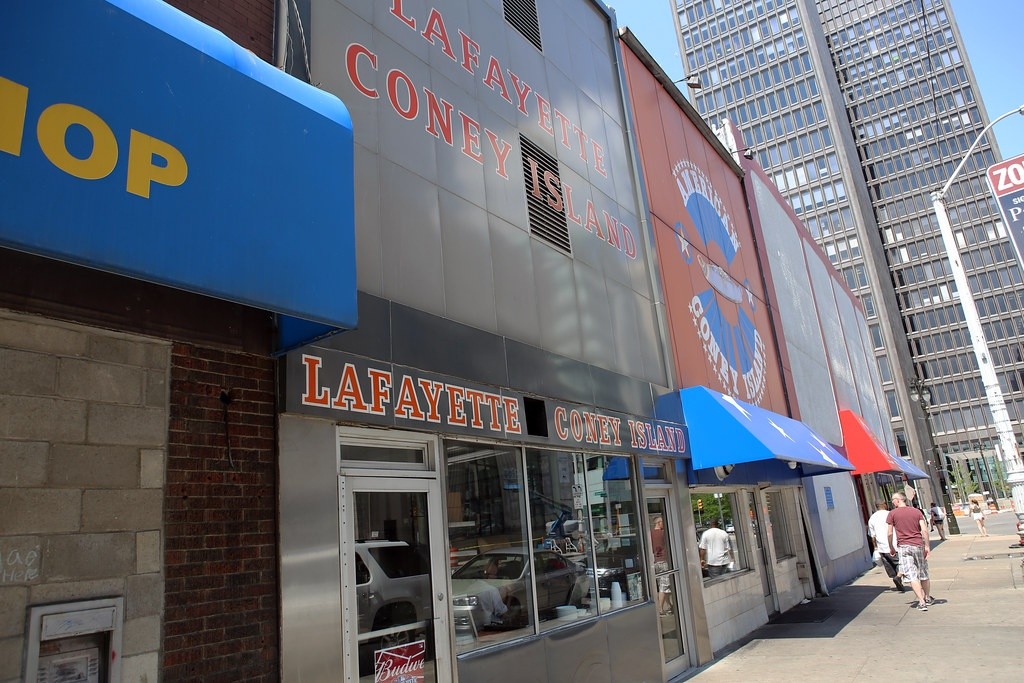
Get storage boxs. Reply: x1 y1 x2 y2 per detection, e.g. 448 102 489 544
448 507 463 522
448 492 461 507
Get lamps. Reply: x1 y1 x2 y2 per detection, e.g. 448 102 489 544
674 77 702 89
730 147 753 160
714 463 736 482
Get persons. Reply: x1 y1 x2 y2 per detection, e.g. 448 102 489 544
699 520 735 581
886 492 934 611
914 503 947 541
466 566 515 635
868 500 908 591
969 499 988 537
650 518 674 618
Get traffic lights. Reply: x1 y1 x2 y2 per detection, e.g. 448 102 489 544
697 499 703 510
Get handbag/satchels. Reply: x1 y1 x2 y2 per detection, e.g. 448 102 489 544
974 512 982 520
729 561 736 571
872 550 883 566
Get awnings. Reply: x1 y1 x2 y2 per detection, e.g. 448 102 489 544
876 451 930 485
681 386 856 488
0 1 360 355
838 409 902 478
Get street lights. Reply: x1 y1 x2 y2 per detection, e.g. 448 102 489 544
929 104 1023 547
906 374 960 535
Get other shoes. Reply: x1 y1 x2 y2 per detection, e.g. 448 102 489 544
893 576 904 591
941 538 945 541
930 529 934 532
981 535 984 537
986 534 989 536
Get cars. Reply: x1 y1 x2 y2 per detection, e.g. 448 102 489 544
695 518 736 541
577 533 640 597
450 546 587 633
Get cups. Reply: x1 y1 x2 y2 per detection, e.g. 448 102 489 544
611 582 623 609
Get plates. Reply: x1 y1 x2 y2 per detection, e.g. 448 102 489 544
556 606 577 618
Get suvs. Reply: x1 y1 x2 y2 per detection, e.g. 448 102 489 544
356 536 432 669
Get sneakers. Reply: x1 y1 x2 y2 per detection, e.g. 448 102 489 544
917 604 927 610
925 595 935 605
659 609 667 617
668 610 672 615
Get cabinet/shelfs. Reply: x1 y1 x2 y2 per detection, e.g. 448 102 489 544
449 521 478 557
